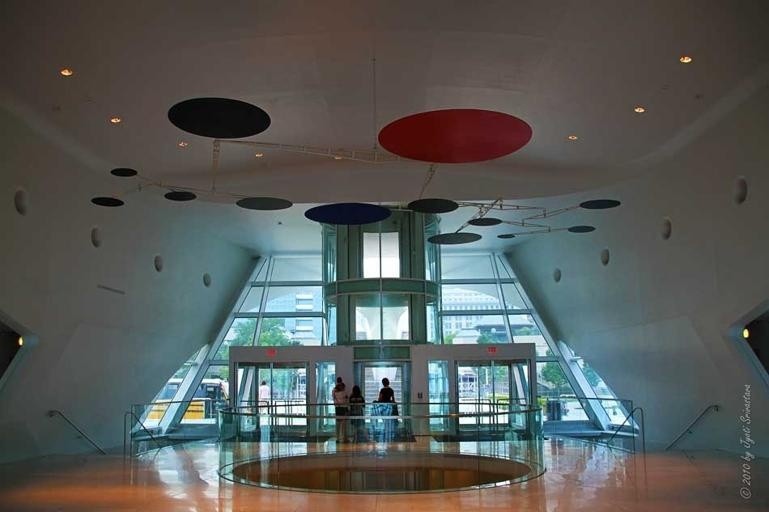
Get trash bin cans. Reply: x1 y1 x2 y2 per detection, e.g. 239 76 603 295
547 401 561 420
210 400 226 419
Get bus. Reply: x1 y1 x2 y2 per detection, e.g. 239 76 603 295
146 379 228 420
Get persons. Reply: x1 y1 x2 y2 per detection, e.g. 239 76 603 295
334 382 350 443
259 380 270 414
337 376 343 384
379 378 395 438
371 399 379 441
349 385 370 442
